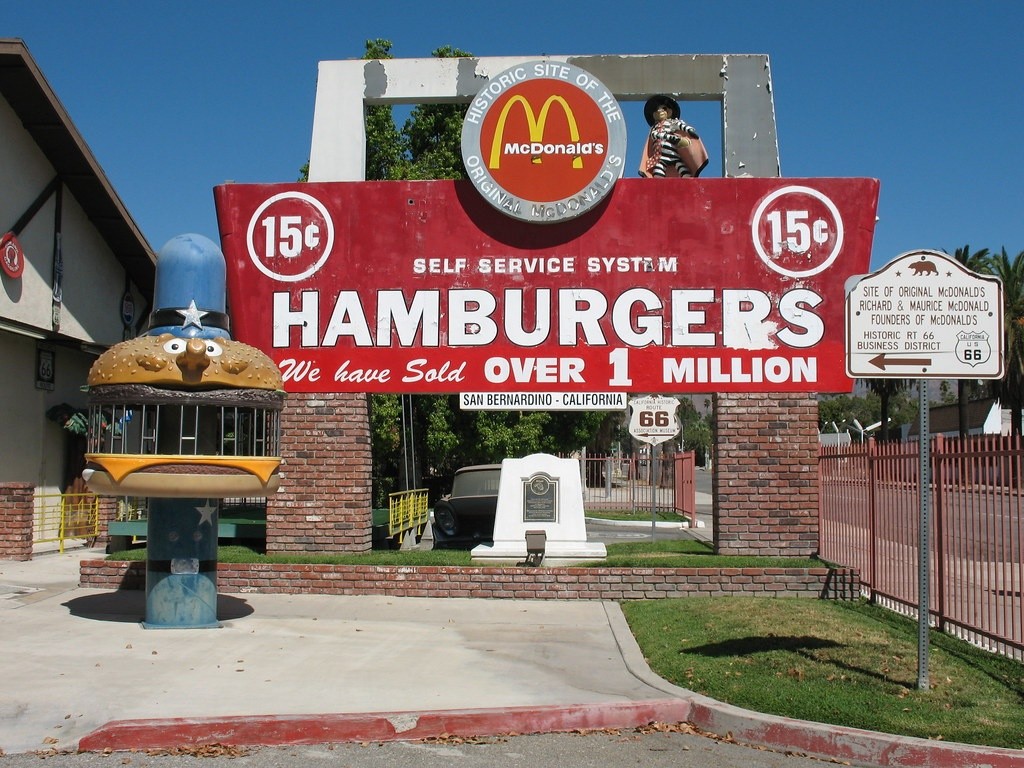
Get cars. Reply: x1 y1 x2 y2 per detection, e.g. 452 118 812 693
431 464 503 551
